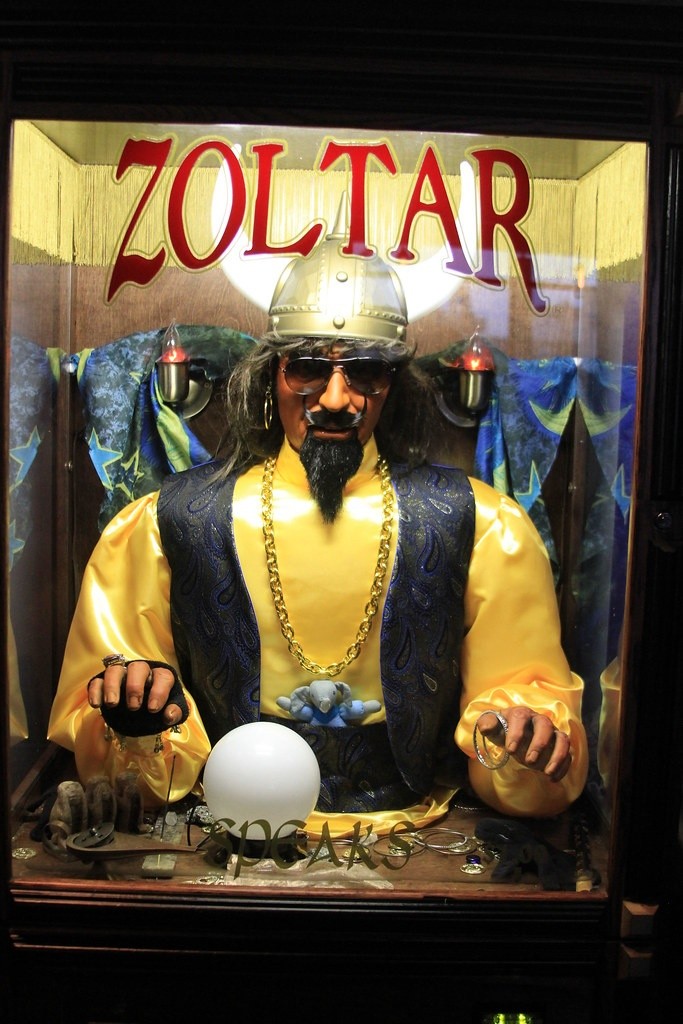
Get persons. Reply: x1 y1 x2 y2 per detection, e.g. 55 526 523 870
47 237 589 819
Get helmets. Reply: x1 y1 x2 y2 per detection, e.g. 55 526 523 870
265 190 408 345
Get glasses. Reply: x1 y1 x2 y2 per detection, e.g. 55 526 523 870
276 357 399 395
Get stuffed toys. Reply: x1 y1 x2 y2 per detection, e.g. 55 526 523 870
275 680 382 727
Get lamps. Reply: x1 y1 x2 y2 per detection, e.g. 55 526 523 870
454 323 499 414
157 320 192 405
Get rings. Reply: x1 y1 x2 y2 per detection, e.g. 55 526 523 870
101 653 127 677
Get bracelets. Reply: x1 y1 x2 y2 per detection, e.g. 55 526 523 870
322 828 477 863
472 709 510 771
103 722 182 754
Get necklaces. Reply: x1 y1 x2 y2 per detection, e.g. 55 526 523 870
260 444 394 680
